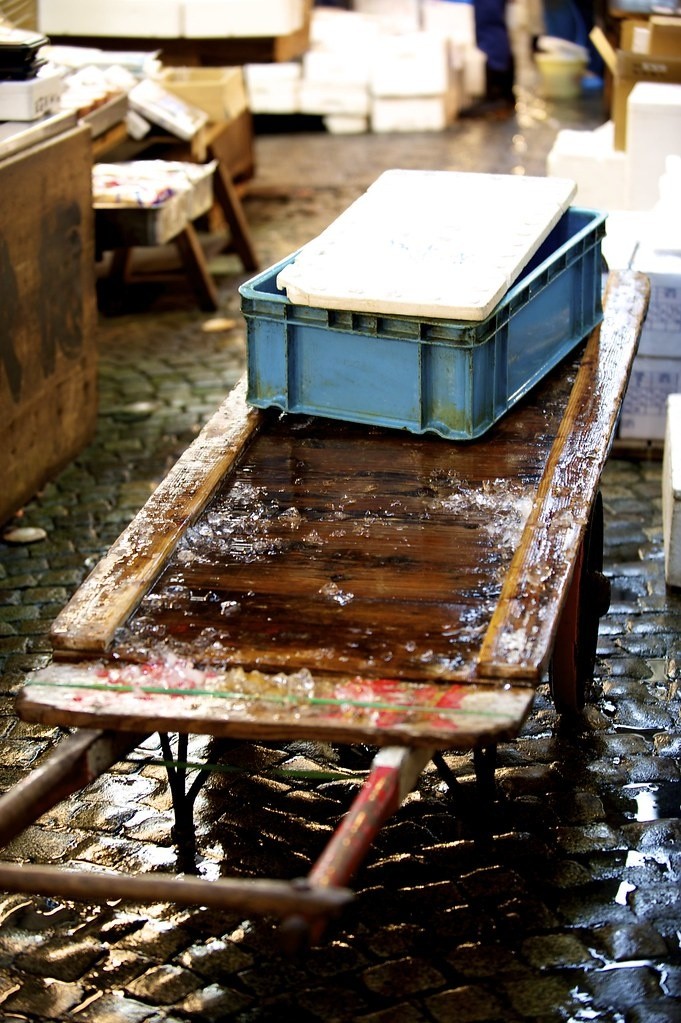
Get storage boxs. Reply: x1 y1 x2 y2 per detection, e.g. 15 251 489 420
1 0 681 443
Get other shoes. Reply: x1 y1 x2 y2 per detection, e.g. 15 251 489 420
457 99 516 122
